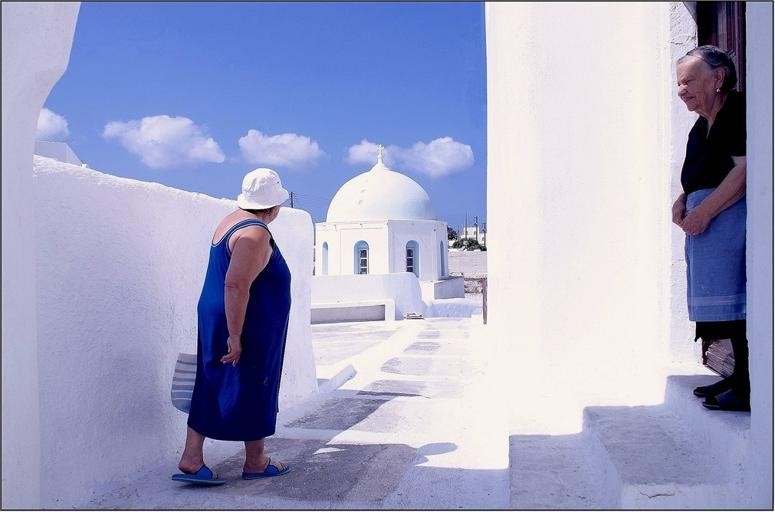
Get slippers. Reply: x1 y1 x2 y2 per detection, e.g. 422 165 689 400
171 463 226 486
241 455 292 480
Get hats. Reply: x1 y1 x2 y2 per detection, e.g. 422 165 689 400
688 45 738 93
237 167 289 210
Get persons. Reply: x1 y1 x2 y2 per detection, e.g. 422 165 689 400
171 168 293 486
670 45 751 414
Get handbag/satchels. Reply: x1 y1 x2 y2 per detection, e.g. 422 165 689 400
170 351 198 415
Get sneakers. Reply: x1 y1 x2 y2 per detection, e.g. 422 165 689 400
693 371 736 398
702 387 751 412
